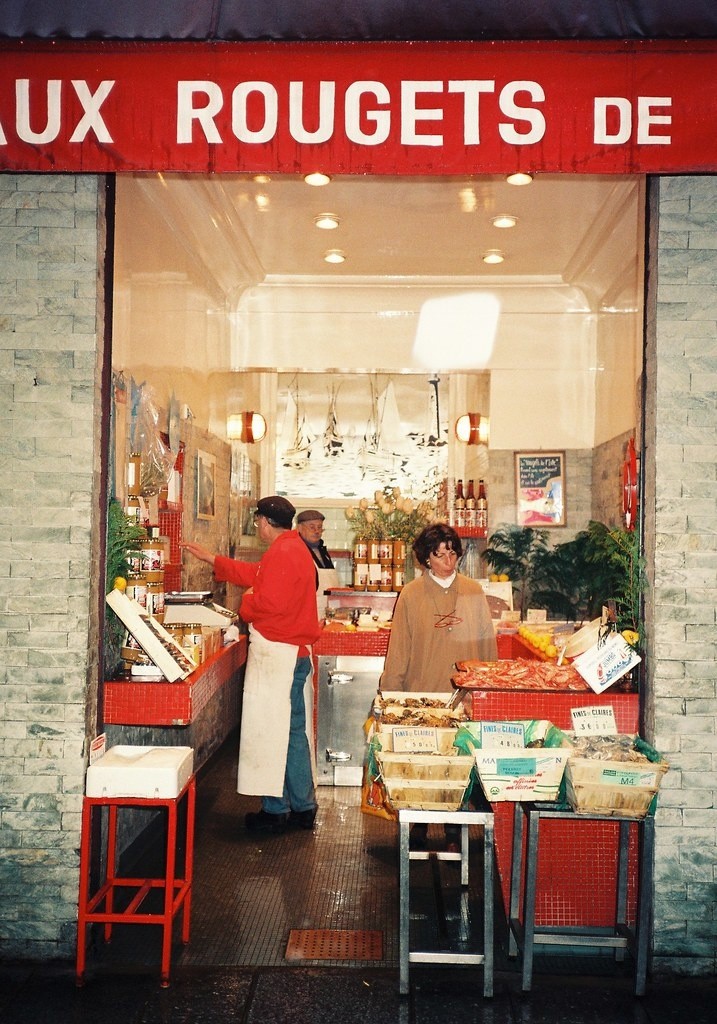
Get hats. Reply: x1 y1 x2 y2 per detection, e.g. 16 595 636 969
257 496 296 523
297 510 325 524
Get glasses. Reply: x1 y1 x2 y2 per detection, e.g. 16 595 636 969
253 520 262 528
433 610 463 628
303 524 325 533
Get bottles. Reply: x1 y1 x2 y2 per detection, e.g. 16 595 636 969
466 480 477 527
476 480 488 529
454 479 466 527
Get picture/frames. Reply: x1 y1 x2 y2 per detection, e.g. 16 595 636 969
513 450 570 529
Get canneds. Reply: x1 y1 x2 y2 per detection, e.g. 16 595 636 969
352 537 406 593
120 452 202 669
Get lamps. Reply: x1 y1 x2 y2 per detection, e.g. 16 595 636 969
227 411 267 444
457 413 488 446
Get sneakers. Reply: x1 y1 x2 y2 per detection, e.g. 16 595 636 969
244 808 287 834
287 805 318 829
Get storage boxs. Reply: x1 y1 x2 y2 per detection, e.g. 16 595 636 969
85 742 198 799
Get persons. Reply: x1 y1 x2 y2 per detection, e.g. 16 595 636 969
179 496 321 833
294 508 340 623
381 524 497 694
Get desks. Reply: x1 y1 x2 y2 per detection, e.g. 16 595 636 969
396 780 497 998
76 774 195 989
509 796 654 992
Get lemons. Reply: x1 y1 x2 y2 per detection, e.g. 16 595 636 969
519 625 639 658
489 573 509 582
113 577 126 591
346 624 356 631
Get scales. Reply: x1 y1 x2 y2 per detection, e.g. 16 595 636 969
162 591 239 626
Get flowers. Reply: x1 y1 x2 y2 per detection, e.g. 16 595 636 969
344 486 441 544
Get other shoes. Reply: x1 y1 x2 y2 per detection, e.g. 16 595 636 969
447 840 460 853
409 825 428 848
